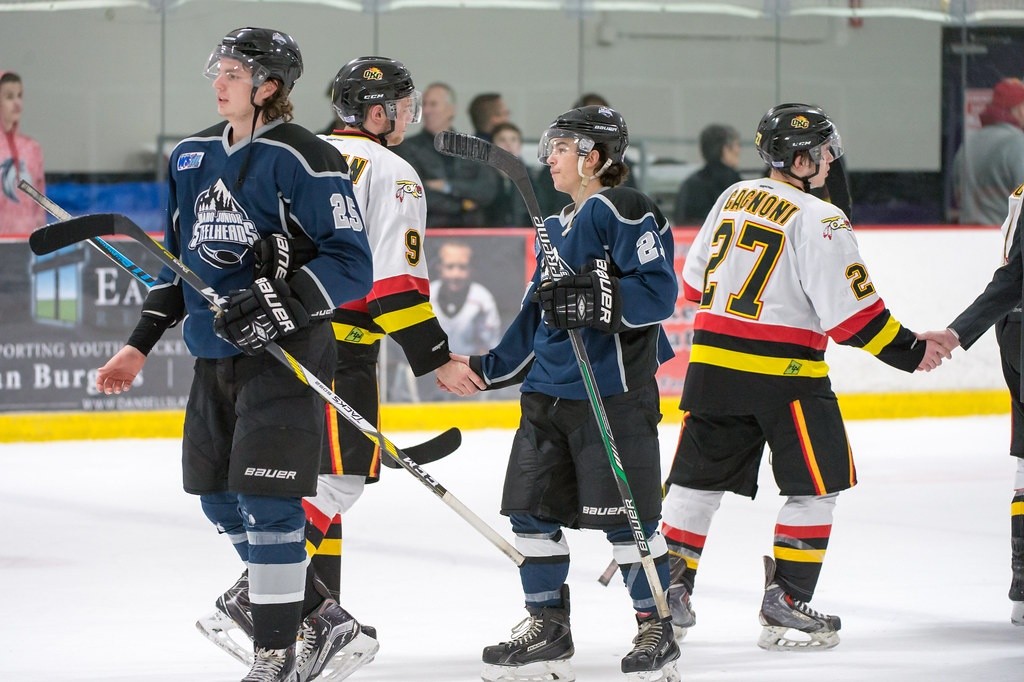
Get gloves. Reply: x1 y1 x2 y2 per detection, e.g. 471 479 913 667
536 259 622 334
209 277 309 356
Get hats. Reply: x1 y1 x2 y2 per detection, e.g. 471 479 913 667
993 78 1024 107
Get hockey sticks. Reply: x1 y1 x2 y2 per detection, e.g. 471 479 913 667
434 130 673 623
17 177 462 468
597 468 673 586
31 214 526 568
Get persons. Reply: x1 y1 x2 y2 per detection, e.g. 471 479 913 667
915 183 1024 626
674 124 743 228
952 77 1024 225
537 94 635 218
96 27 381 682
436 104 681 682
195 57 488 667
662 103 953 652
397 82 534 229
428 241 501 356
0 70 46 235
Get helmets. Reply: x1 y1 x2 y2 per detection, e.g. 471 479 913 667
324 57 414 128
219 29 303 102
754 103 833 168
547 105 628 166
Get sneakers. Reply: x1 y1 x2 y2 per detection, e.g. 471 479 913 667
283 575 380 682
241 641 296 682
194 569 257 666
481 584 575 681
757 554 840 650
620 586 682 682
1009 540 1024 623
668 550 699 641
